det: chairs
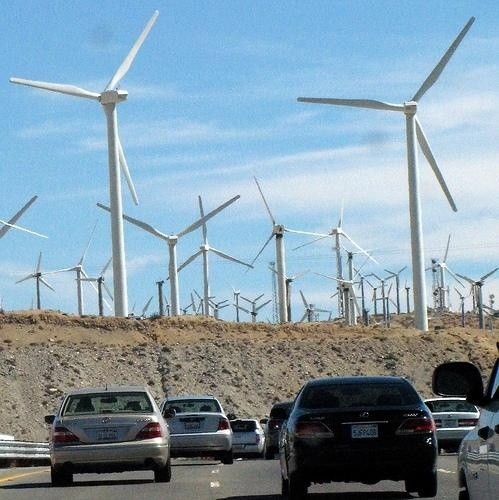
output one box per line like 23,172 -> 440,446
170,406 -> 181,412
74,403 -> 94,412
124,400 -> 142,410
200,405 -> 211,411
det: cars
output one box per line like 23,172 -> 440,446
260,357 -> 498,499
44,390 -> 265,484
